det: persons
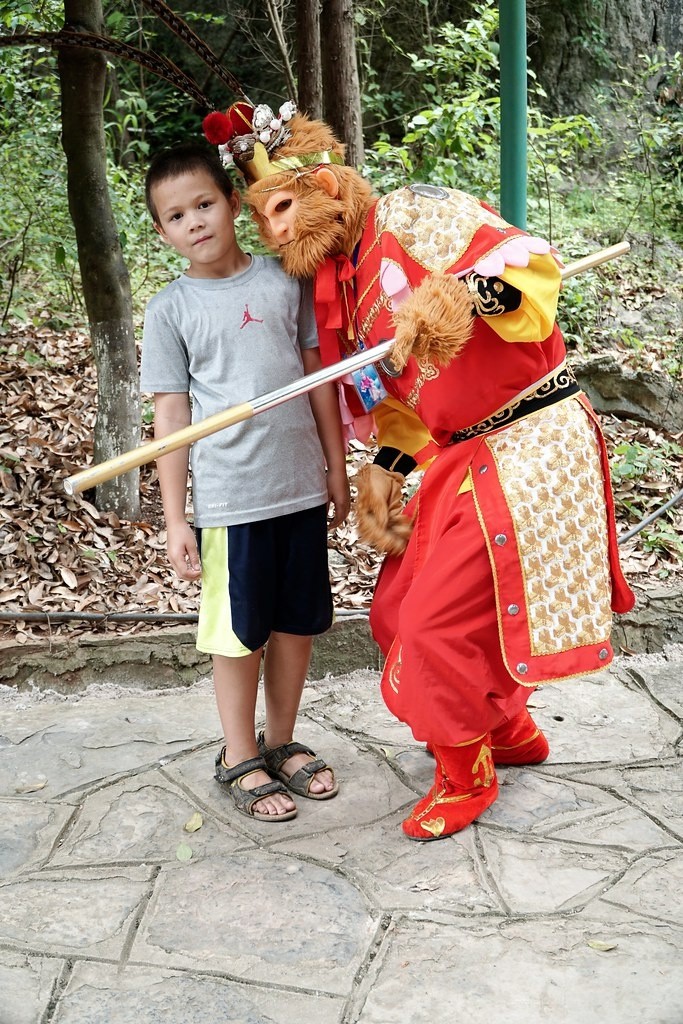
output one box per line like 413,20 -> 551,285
140,142 -> 351,822
203,104 -> 636,843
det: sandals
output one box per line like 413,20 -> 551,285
256,729 -> 339,801
214,745 -> 298,822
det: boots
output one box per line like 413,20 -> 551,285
403,733 -> 499,839
425,702 -> 550,768
